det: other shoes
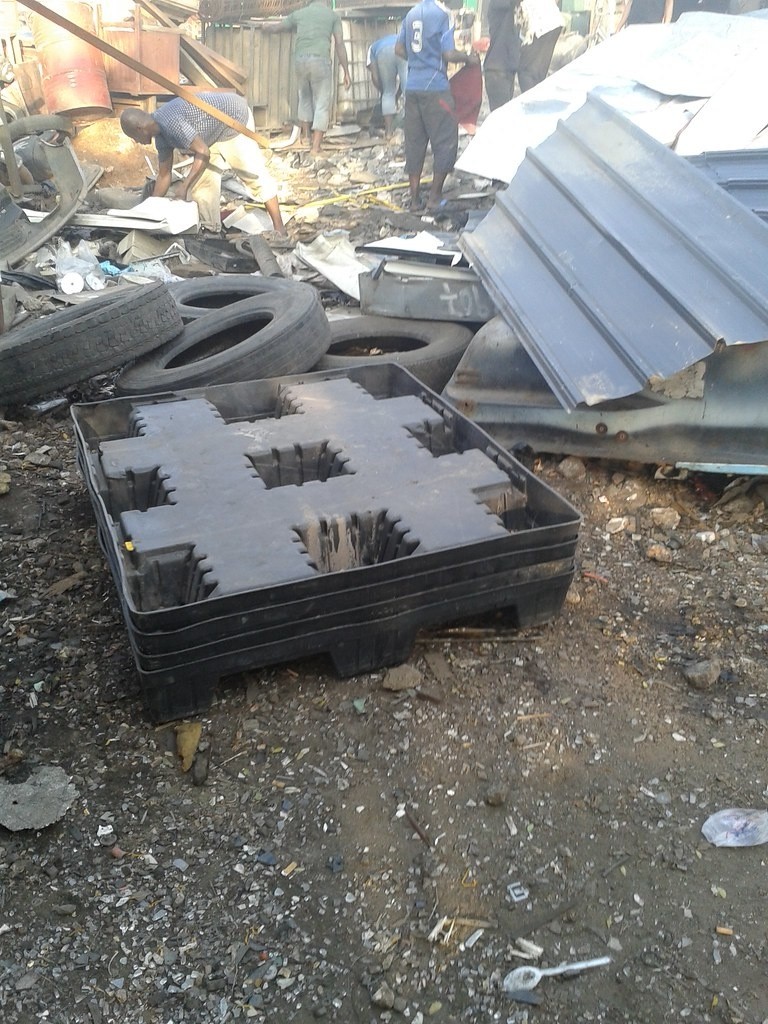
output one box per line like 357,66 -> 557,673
300,134 -> 313,146
309,150 -> 331,159
369,126 -> 391,140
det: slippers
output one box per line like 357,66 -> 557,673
426,200 -> 459,214
409,197 -> 428,211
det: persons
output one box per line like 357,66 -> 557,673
120,92 -> 283,230
365,36 -> 407,128
261,0 -> 352,150
615,0 -> 730,34
485,1 -> 562,111
395,0 -> 482,210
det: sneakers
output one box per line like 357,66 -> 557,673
267,230 -> 296,248
196,233 -> 223,241
